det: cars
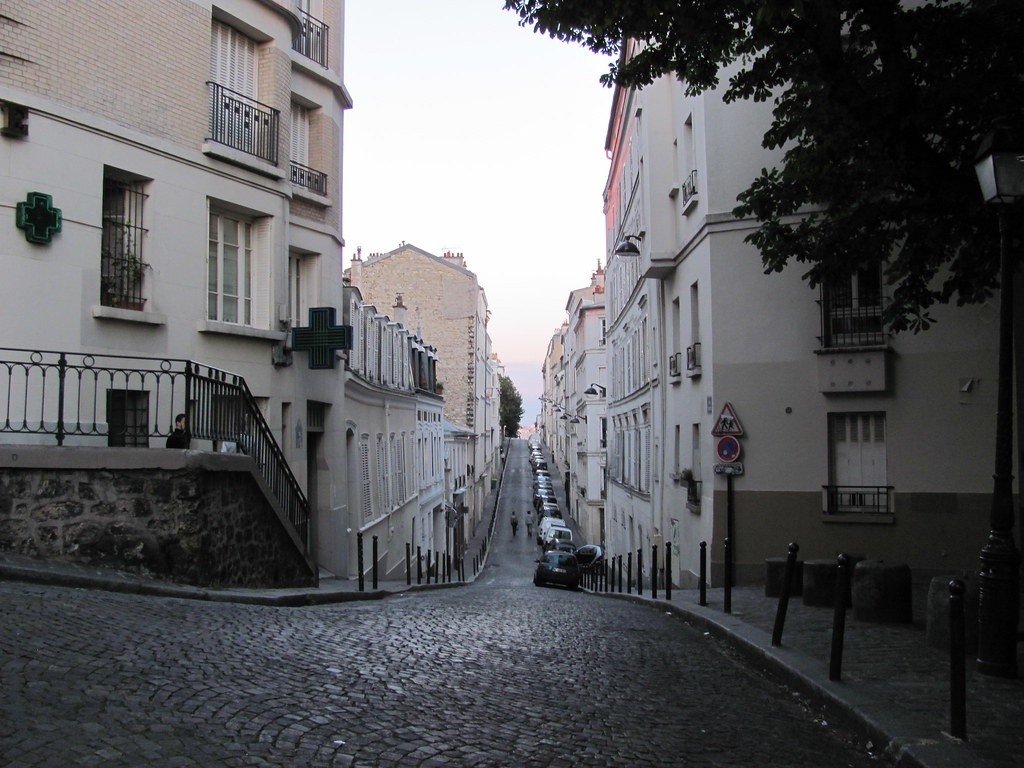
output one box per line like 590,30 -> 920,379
532,549 -> 584,592
573,544 -> 605,568
528,432 -> 577,554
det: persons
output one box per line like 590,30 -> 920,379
510,511 -> 518,536
525,510 -> 535,537
166,414 -> 189,450
221,414 -> 254,455
